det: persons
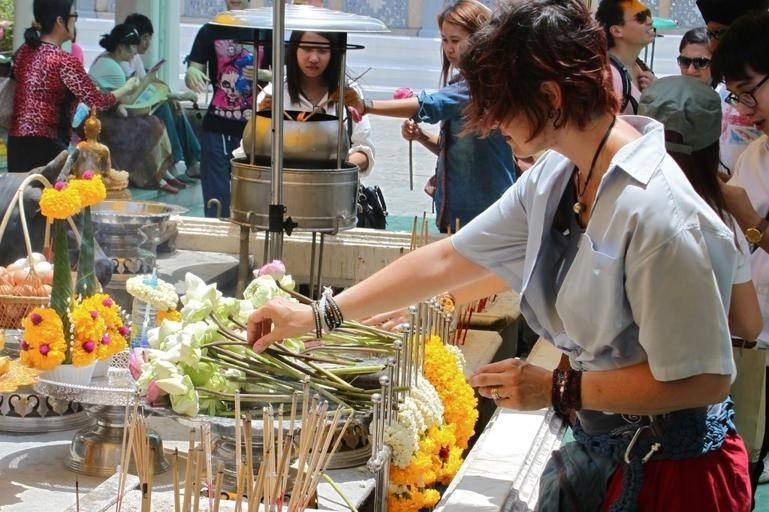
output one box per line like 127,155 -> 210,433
247,1 -> 752,511
5,0 -> 145,174
121,12 -> 205,186
83,23 -> 185,194
593,0 -> 658,117
258,30 -> 377,174
334,0 -> 517,238
638,0 -> 769,512
182,1 -> 281,221
73,104 -> 110,179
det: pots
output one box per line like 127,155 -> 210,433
242,110 -> 351,168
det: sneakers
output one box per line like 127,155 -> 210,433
169,178 -> 186,188
164,183 -> 176,192
186,171 -> 200,179
177,175 -> 196,183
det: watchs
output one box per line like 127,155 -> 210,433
744,219 -> 768,244
362,98 -> 373,114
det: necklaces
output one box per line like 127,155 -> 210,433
571,115 -> 618,215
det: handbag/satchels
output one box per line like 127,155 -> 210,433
357,182 -> 387,229
534,437 -> 623,512
424,176 -> 437,196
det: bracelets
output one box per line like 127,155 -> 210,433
553,352 -> 581,410
312,285 -> 343,338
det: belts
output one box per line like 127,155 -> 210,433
732,338 -> 760,348
580,414 -> 664,436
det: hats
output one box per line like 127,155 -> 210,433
639,76 -> 722,154
696,0 -> 768,27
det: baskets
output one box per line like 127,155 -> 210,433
1,174 -> 101,328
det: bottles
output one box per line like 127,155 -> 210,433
130,274 -> 164,353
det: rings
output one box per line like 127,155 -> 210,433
491,387 -> 502,402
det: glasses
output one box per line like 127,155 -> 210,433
705,28 -> 726,39
121,28 -> 140,43
633,9 -> 651,25
67,11 -> 78,21
725,76 -> 768,108
677,56 -> 710,70
